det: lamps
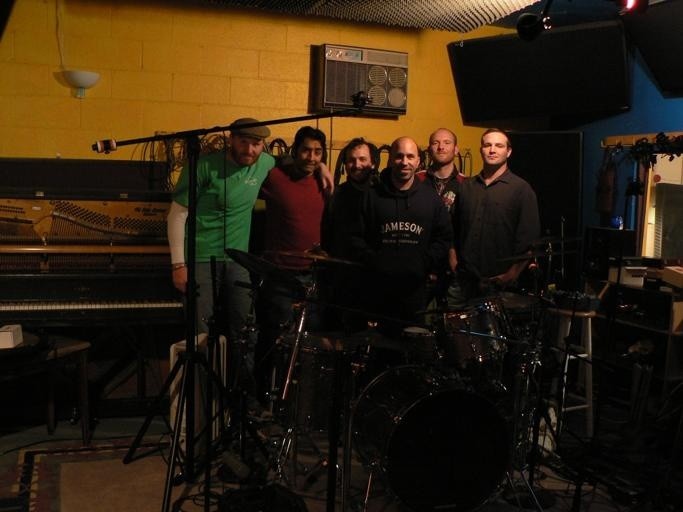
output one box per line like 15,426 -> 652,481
61,70 -> 100,99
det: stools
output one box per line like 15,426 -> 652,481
0,335 -> 92,448
533,308 -> 596,435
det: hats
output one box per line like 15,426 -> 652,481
229,118 -> 271,140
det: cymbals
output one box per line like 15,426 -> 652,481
225,249 -> 302,284
264,250 -> 353,264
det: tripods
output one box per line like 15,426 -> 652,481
250,249 -> 599,512
92,110 -> 357,512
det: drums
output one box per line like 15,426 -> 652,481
400,326 -> 436,364
350,365 -> 510,503
443,298 -> 501,363
295,349 -> 350,435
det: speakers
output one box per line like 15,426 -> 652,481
446,20 -> 631,127
621,0 -> 683,98
506,131 -> 585,240
321,43 -> 409,115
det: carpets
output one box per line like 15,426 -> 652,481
6,431 -> 630,511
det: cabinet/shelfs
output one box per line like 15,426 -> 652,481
583,267 -> 683,415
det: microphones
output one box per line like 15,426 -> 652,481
350,91 -> 373,109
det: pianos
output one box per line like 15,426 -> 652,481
0,158 -> 183,311
